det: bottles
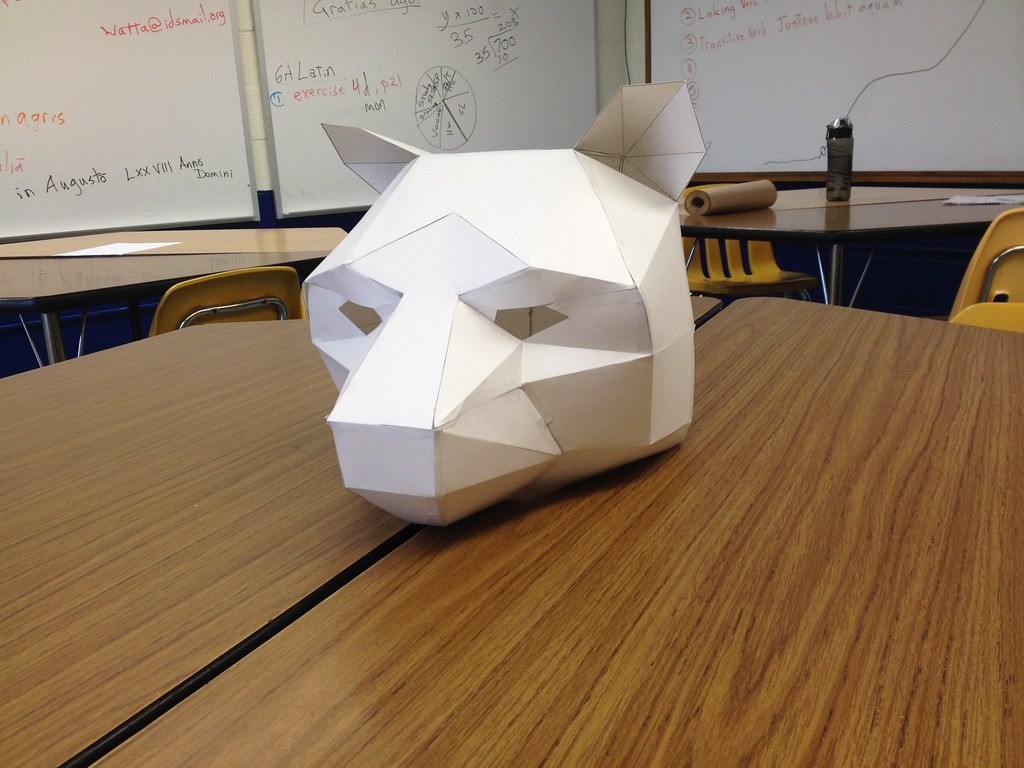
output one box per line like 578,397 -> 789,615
825,117 -> 854,201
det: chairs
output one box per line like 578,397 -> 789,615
673,176 -> 816,302
949,203 -> 1024,321
149,264 -> 299,340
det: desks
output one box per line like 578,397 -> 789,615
1,222 -> 352,374
2,289 -> 1024,767
676,180 -> 1024,321
